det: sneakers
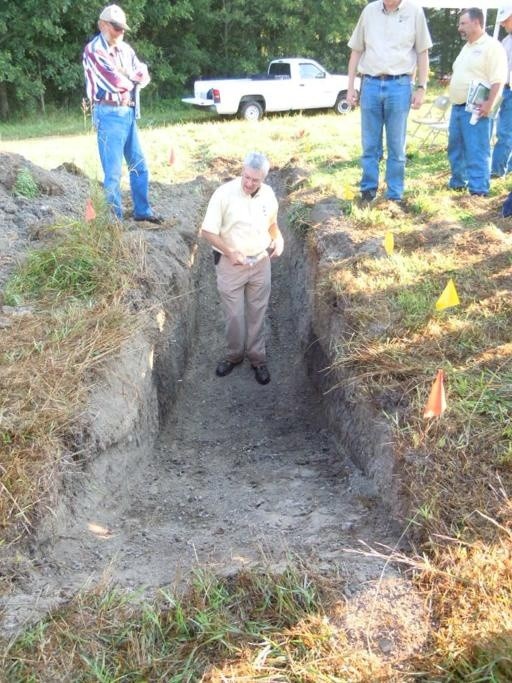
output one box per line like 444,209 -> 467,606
359,191 -> 375,210
134,211 -> 165,224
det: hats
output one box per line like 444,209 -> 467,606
496,0 -> 512,22
99,4 -> 131,30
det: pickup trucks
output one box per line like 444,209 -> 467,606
180,55 -> 362,120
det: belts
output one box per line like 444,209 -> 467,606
363,74 -> 407,80
100,99 -> 136,106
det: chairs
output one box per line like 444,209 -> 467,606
411,95 -> 451,147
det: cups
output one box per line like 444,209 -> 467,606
468,110 -> 481,126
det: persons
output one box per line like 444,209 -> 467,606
344,0 -> 434,202
199,151 -> 285,385
83,3 -> 166,224
441,7 -> 507,194
487,7 -> 512,178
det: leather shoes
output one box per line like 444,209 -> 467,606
216,359 -> 243,376
252,365 -> 269,385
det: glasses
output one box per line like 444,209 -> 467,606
110,24 -> 122,31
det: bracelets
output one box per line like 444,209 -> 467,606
415,84 -> 424,88
